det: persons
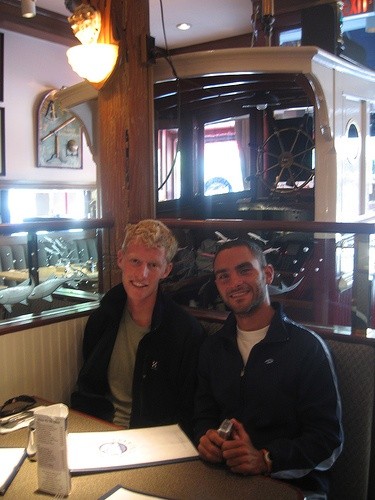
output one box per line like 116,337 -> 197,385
193,239 -> 344,476
71,219 -> 207,438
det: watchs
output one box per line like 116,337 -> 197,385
262,450 -> 272,474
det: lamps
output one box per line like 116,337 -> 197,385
21,0 -> 36,19
66,0 -> 119,83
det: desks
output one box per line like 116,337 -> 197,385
0,264 -> 101,289
0,395 -> 306,500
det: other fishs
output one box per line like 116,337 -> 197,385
0,273 -> 76,313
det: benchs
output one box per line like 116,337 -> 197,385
181,306 -> 375,500
0,229 -> 100,291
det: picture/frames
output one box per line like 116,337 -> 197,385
36,88 -> 84,169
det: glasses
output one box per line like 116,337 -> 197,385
0,395 -> 37,417
